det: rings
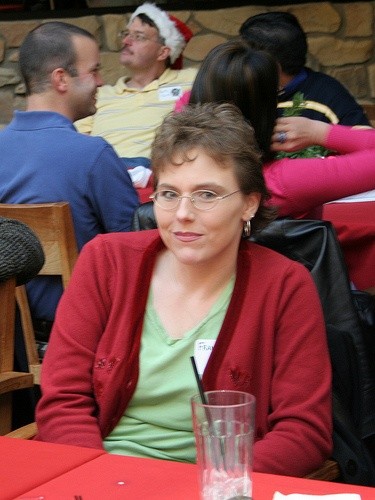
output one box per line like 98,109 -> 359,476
278,131 -> 286,144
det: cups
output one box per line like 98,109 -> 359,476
191,390 -> 256,500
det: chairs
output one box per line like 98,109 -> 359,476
0,200 -> 375,486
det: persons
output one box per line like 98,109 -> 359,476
0,3 -> 375,287
35,101 -> 332,479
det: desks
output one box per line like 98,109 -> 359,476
0,434 -> 375,500
128,166 -> 375,295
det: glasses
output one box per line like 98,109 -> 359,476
148,189 -> 250,210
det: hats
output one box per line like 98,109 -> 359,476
129,2 -> 193,69
